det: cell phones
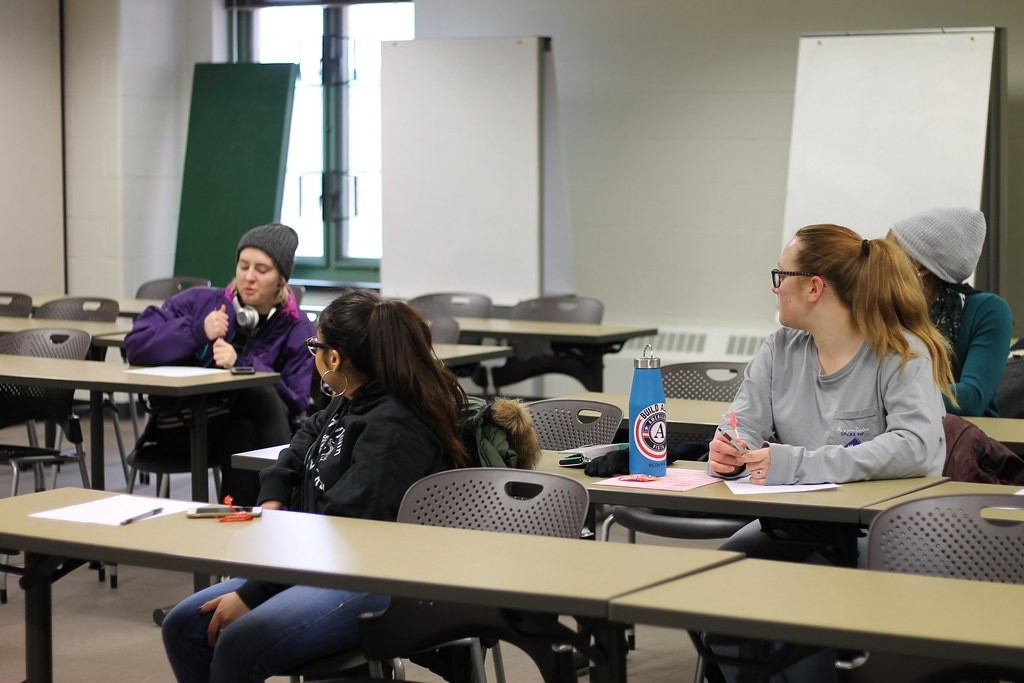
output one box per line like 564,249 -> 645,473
559,453 -> 583,465
187,506 -> 263,519
231,366 -> 254,375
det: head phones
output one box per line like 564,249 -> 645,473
232,290 -> 278,329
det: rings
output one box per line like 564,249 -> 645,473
757,470 -> 762,478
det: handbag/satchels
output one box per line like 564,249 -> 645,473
132,374 -> 258,472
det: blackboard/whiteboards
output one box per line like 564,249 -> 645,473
778,26 -> 996,302
380,35 -> 542,307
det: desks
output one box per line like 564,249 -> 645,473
860,481 -> 1024,528
231,439 -> 951,676
0,315 -> 134,470
0,291 -> 657,394
0,354 -> 281,626
0,298 -> 1024,565
1,484 -> 745,683
517,391 -> 1024,485
91,331 -> 514,397
608,558 -> 1024,683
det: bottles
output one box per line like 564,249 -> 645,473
628,344 -> 668,477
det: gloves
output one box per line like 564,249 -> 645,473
583,442 -> 681,477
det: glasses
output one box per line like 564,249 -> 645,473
306,335 -> 334,358
771,268 -> 827,291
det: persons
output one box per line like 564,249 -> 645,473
163,288 -> 468,683
708,224 -> 960,683
886,205 -> 1014,418
123,223 -> 316,505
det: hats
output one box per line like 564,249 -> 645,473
236,223 -> 299,280
890,206 -> 988,283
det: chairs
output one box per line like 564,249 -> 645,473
0,274 -> 1024,650
291,466 -> 589,683
836,493 -> 1023,683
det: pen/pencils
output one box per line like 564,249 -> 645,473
718,428 -> 746,453
730,410 -> 739,438
215,307 -> 221,339
119,508 -> 164,526
